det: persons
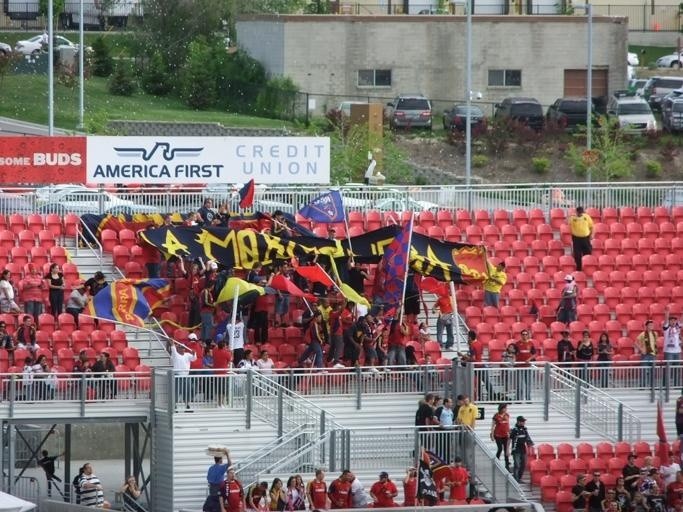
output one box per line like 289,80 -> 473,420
0,196 -> 682,511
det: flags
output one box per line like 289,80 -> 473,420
238,177 -> 254,208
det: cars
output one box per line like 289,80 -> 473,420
14,32 -> 94,63
0,41 -> 13,57
626,46 -> 683,136
336,99 -> 363,117
439,103 -> 487,133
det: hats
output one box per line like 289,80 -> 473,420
516,416 -> 525,421
563,275 -> 571,280
210,263 -> 218,269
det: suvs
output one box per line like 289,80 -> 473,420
488,94 -> 543,132
543,94 -> 599,130
383,92 -> 434,133
605,89 -> 659,138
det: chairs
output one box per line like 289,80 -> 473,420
365,496 -> 487,511
0,205 -> 682,387
525,440 -> 682,512
